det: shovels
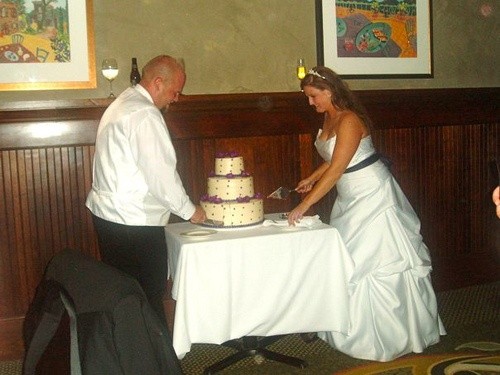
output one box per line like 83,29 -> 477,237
267,187 -> 295,200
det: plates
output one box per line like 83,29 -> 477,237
179,229 -> 216,241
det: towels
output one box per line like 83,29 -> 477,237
262,215 -> 322,229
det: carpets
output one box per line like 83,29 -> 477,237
330,353 -> 500,374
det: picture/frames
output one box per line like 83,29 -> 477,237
0,0 -> 98,92
315,0 -> 435,80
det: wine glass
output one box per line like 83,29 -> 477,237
102,59 -> 118,97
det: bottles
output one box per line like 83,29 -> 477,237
130,57 -> 140,86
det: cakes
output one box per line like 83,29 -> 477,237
199,151 -> 265,227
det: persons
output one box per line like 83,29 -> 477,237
288,64 -> 446,363
86,55 -> 207,343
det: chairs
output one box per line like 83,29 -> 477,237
37,250 -> 163,374
